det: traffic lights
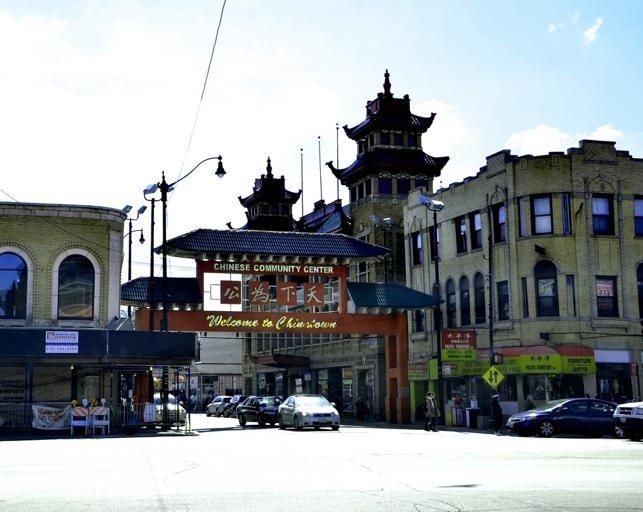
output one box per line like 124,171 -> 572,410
493,352 -> 503,365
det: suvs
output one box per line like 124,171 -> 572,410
612,402 -> 643,440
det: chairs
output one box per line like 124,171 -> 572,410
68,401 -> 112,438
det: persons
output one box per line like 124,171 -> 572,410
524,394 -> 535,411
356,396 -> 365,421
429,392 -> 438,432
424,392 -> 438,432
491,395 -> 504,435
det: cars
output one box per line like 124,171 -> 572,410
504,398 -> 620,438
141,391 -> 187,429
205,388 -> 340,431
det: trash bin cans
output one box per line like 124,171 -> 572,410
466,407 -> 480,428
477,413 -> 489,429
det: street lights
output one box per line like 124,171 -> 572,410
369,212 -> 393,284
413,194 -> 446,425
120,153 -> 226,427
